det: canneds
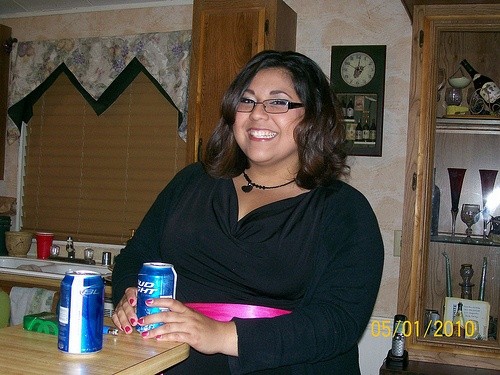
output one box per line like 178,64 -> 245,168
58,270 -> 105,354
136,262 -> 177,334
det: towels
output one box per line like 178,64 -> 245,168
9,286 -> 56,326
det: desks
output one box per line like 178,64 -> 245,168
0,323 -> 189,375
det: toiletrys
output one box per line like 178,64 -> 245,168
129,228 -> 135,240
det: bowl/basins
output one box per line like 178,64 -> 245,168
5,231 -> 34,256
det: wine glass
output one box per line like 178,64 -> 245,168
447,167 -> 467,237
478,168 -> 498,239
460,204 -> 480,239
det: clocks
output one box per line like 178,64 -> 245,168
329,45 -> 387,157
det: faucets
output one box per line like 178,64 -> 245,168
66,236 -> 76,259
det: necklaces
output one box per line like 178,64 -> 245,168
242,171 -> 296,193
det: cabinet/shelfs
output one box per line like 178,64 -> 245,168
398,0 -> 500,371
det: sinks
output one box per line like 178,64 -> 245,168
0,256 -> 109,280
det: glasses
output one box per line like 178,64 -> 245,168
236,99 -> 303,113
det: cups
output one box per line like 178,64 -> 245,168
36,232 -> 55,260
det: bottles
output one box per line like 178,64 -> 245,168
430,310 -> 441,334
460,58 -> 500,114
355,118 -> 363,141
346,99 -> 355,119
450,302 -> 465,339
369,118 -> 376,142
427,168 -> 441,237
340,99 -> 347,119
362,118 -> 370,142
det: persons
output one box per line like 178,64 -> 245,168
110,50 -> 385,375
